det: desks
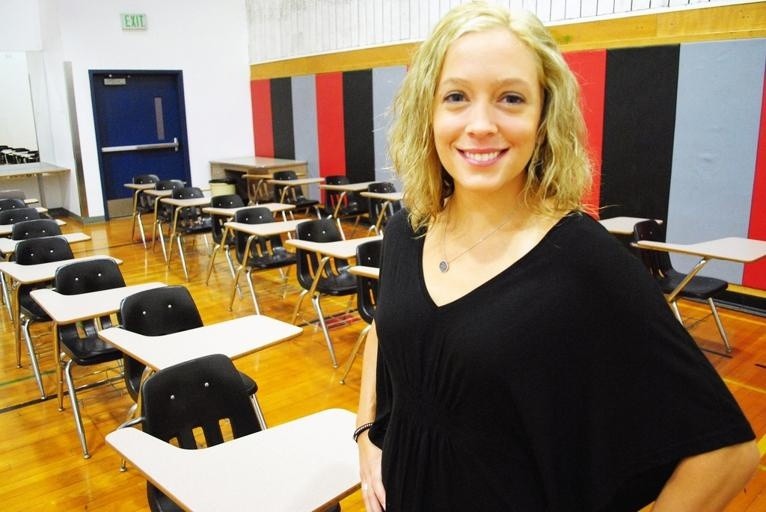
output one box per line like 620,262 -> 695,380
0,161 -> 70,207
208,157 -> 309,200
628,236 -> 765,304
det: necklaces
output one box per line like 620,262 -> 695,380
436,192 -> 529,275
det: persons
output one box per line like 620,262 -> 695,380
349,0 -> 761,512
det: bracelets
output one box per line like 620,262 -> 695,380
351,423 -> 377,441
